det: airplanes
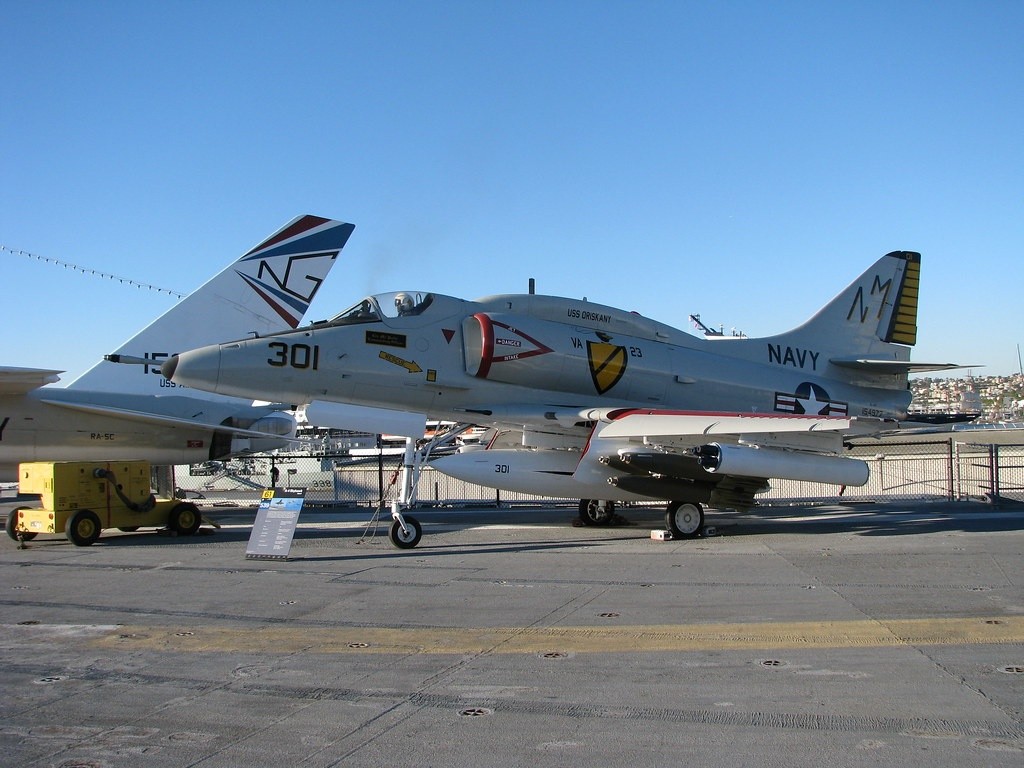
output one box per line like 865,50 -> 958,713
0,212 -> 1023,550
